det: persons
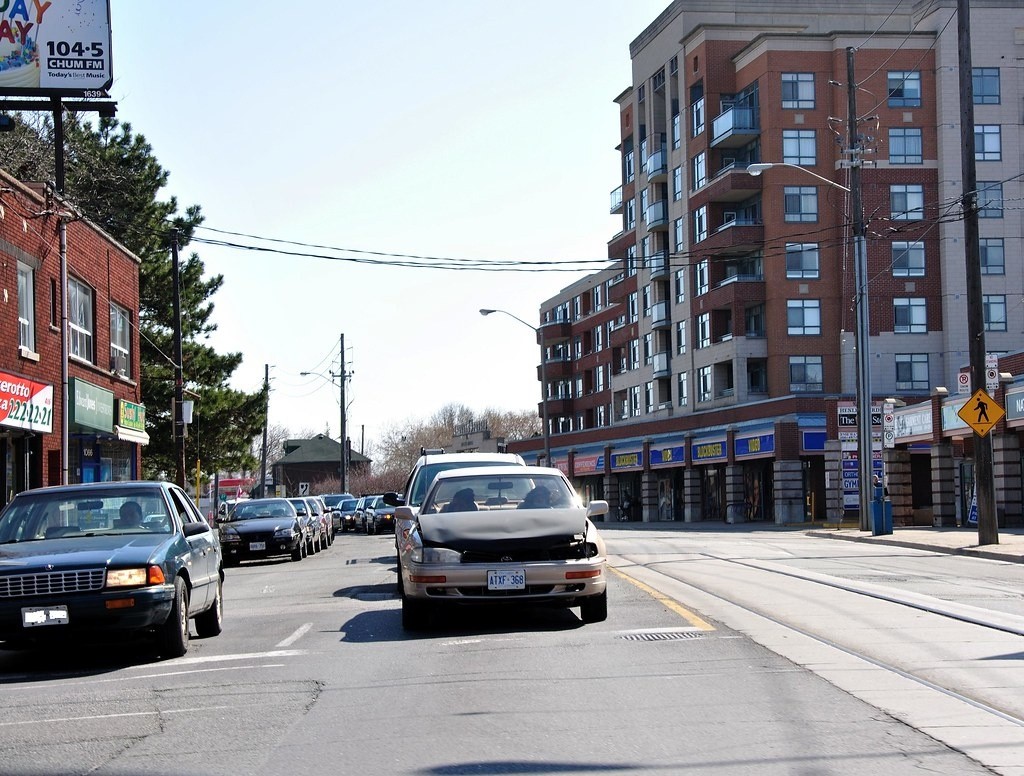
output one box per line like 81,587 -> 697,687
621,489 -> 631,521
119,501 -> 143,528
440,487 -> 552,513
873,474 -> 888,496
748,473 -> 764,518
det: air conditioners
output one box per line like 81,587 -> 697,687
110,357 -> 126,370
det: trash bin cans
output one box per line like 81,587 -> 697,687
869,487 -> 893,536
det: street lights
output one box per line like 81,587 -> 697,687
478,309 -> 551,469
300,371 -> 347,495
747,161 -> 873,529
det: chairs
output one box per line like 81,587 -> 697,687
440,502 -> 478,512
141,521 -> 166,532
241,513 -> 256,519
368,502 -> 372,507
45,527 -> 81,538
378,503 -> 386,509
272,510 -> 284,516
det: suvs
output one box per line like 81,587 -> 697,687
382,452 -> 561,548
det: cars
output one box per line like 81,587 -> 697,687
0,478 -> 226,664
216,491 -> 408,567
395,465 -> 610,629
